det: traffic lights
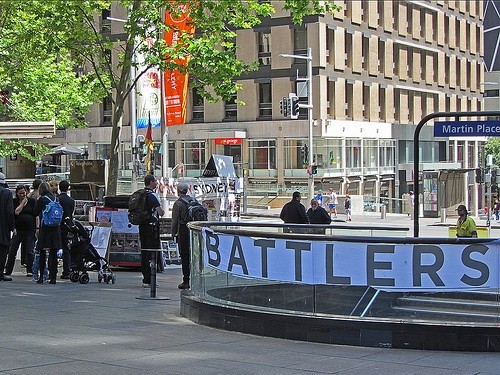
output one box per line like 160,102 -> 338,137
291,96 -> 300,119
280,96 -> 287,117
312,165 -> 318,174
475,168 -> 481,183
139,152 -> 147,159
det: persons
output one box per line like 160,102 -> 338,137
280,191 -> 351,234
0,172 -> 76,284
455,205 -> 478,238
408,192 -> 415,221
485,199 -> 500,225
138,175 -> 165,288
172,182 -> 198,289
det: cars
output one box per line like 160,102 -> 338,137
164,164 -> 203,178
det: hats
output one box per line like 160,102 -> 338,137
454,204 -> 466,211
0,172 -> 6,184
176,183 -> 189,192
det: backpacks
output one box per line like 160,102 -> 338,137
180,196 -> 208,221
128,189 -> 156,225
41,195 -> 64,227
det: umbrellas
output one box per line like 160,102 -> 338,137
46,141 -> 84,172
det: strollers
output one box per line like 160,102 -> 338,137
61,217 -> 116,285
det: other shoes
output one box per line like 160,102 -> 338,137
36,279 -> 43,284
0,276 -> 13,281
60,273 -> 70,279
3,271 -> 10,275
142,282 -> 159,288
178,281 -> 190,289
49,280 -> 56,284
